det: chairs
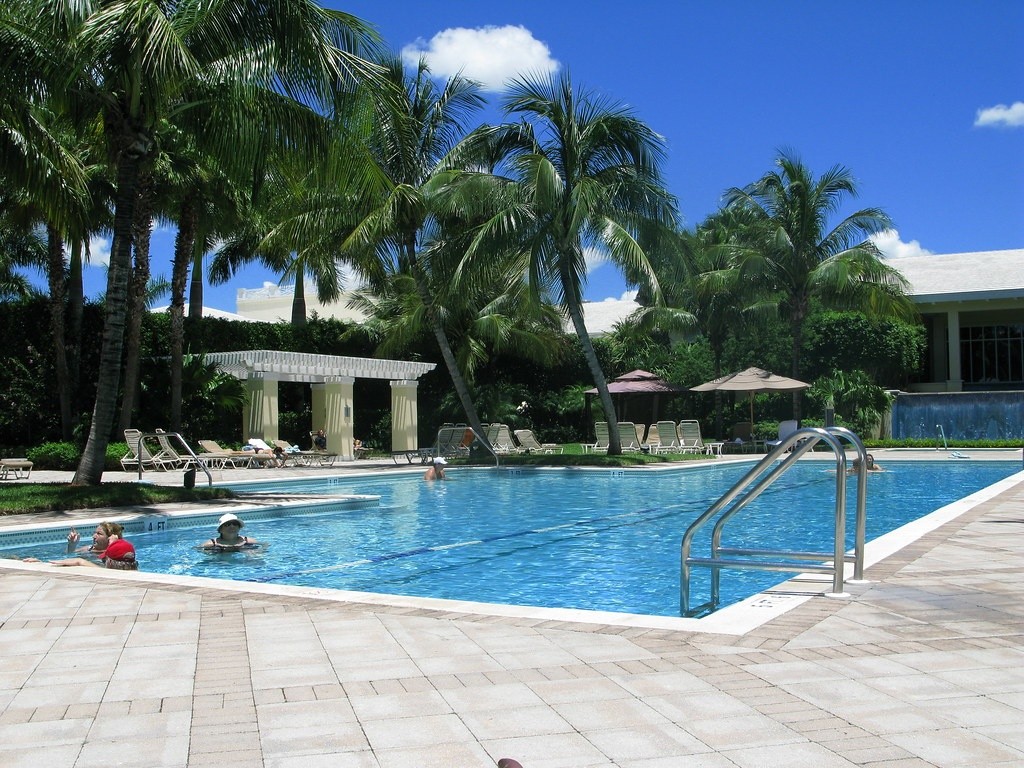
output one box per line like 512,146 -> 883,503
635,424 -> 645,446
249,438 -> 321,467
418,423 -> 518,465
593,421 -> 609,454
655,421 -> 679,455
271,440 -> 337,467
679,420 -> 706,455
121,429 -> 170,473
309,431 -> 320,452
514,430 -> 564,455
725,423 -> 751,455
156,429 -> 229,471
354,448 -> 374,460
646,423 -> 660,454
198,440 -> 270,471
766,421 -> 798,452
617,422 -> 641,453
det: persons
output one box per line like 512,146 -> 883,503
424,457 -> 448,480
315,428 -> 362,451
67,522 -> 124,552
202,513 -> 254,545
252,446 -> 288,468
23,539 -> 138,570
852,454 -> 879,471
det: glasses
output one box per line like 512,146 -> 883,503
222,521 -> 241,527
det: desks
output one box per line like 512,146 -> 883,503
580,444 -> 596,454
391,450 -> 419,464
752,439 -> 768,453
705,443 -> 724,455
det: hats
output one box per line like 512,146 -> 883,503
434,457 -> 448,464
216,513 -> 244,531
98,539 -> 135,561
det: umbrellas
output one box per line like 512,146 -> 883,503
582,369 -> 688,422
688,367 -> 812,439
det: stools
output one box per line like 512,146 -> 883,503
0,459 -> 33,480
